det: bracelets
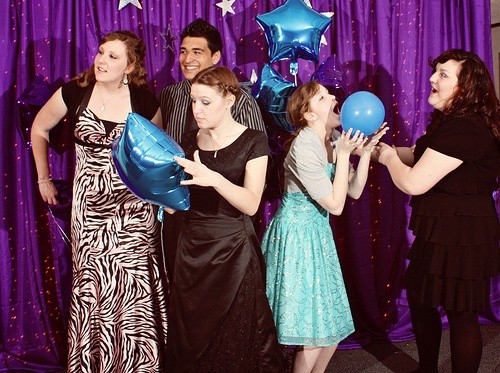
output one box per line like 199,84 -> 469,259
37,178 -> 52,183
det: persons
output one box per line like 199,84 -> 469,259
31,30 -> 170,373
165,67 -> 283,373
161,17 -> 267,142
261,82 -> 389,373
350,49 -> 500,373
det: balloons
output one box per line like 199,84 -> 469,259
255,0 -> 333,64
340,92 -> 385,136
255,64 -> 297,133
113,113 -> 190,210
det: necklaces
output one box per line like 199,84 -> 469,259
208,120 -> 236,158
96,83 -> 124,110
